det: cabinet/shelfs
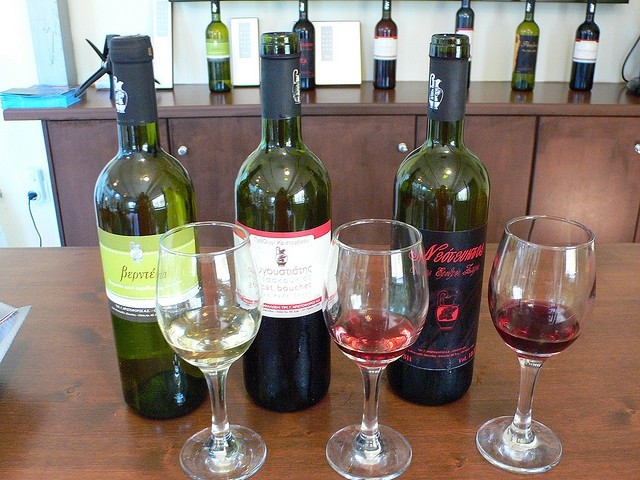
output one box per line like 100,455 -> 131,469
0,84 -> 640,246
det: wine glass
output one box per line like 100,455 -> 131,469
157,222 -> 267,476
324,218 -> 430,480
477,213 -> 598,473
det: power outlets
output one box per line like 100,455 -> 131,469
26,169 -> 46,205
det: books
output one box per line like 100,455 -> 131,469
0,84 -> 81,108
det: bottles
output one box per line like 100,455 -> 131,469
234,31 -> 334,412
455,0 -> 474,88
569,0 -> 600,92
390,33 -> 489,403
205,1 -> 233,93
511,1 -> 540,92
293,0 -> 316,91
372,1 -> 397,87
95,34 -> 207,416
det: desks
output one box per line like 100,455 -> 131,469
0,241 -> 640,480
